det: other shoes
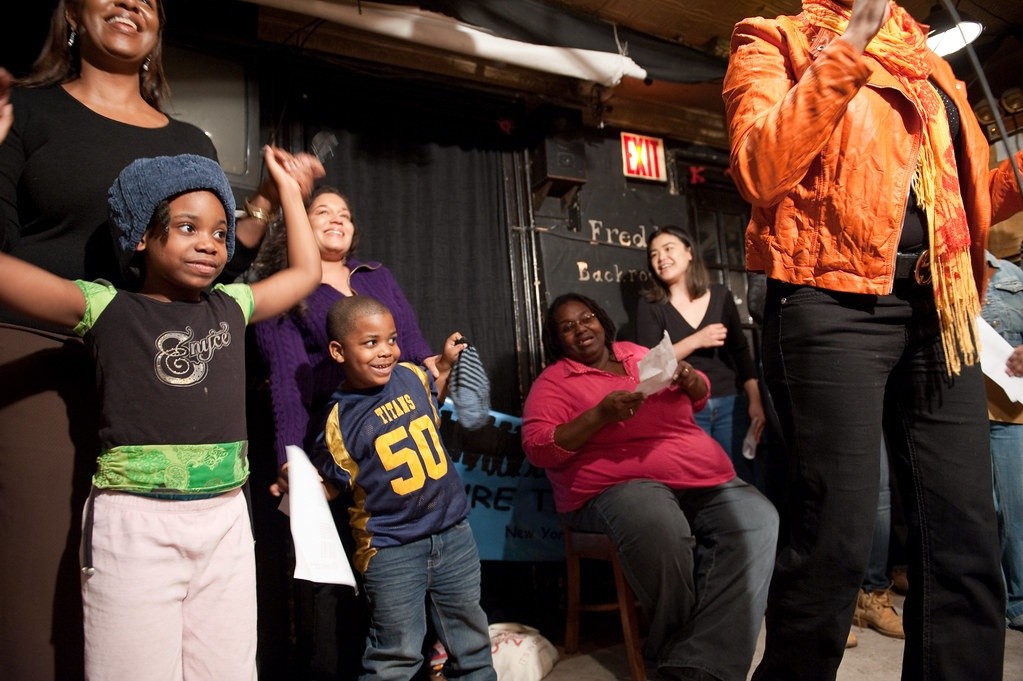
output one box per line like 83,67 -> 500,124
1008,623 -> 1022,629
890,570 -> 908,592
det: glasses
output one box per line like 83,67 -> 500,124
559,313 -> 598,336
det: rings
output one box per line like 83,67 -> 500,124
685,369 -> 689,373
629,408 -> 634,416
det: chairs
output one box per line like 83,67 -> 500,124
559,513 -> 697,680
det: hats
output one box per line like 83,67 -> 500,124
448,345 -> 490,430
106,152 -> 236,277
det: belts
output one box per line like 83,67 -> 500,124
895,250 -> 933,286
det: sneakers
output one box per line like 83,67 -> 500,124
845,633 -> 856,645
853,590 -> 905,639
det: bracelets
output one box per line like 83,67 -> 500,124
243,196 -> 270,223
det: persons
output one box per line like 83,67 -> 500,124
723,0 -> 1023,681
520,293 -> 780,681
637,225 -> 766,463
0,0 -> 328,681
251,186 -> 499,681
